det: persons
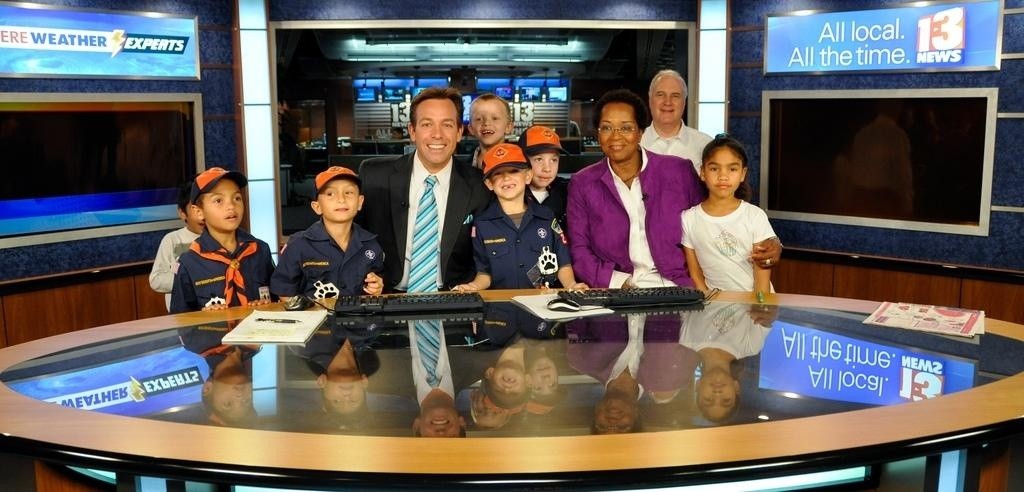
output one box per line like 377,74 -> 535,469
287,304 -> 780,440
169,167 -> 280,313
449,145 -> 590,295
831,101 -> 915,218
640,68 -> 715,177
565,89 -> 784,290
679,133 -> 783,294
354,87 -> 494,293
519,125 -> 571,288
149,181 -> 206,315
177,321 -> 264,429
465,94 -> 515,170
268,166 -> 384,311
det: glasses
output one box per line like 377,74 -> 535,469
598,126 -> 637,135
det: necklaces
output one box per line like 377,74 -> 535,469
620,151 -> 643,183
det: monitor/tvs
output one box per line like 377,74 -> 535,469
377,142 -> 404,154
354,85 -> 569,128
759,86 -> 999,239
558,139 -> 580,155
457,140 -> 478,154
0,91 -> 208,252
349,141 -> 376,154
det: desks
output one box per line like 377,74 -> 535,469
0,287 -> 1024,491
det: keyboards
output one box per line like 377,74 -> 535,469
333,291 -> 487,313
558,285 -> 707,307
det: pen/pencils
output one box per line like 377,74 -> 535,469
254,317 -> 301,323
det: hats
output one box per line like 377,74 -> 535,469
484,391 -> 525,414
525,382 -> 568,414
520,125 -> 571,156
191,167 -> 248,205
314,166 -> 360,200
202,397 -> 257,426
482,142 -> 532,179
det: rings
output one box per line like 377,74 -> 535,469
766,259 -> 772,265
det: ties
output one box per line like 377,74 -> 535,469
408,176 -> 438,293
414,318 -> 441,390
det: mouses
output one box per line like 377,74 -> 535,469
548,298 -> 581,312
284,294 -> 315,312
